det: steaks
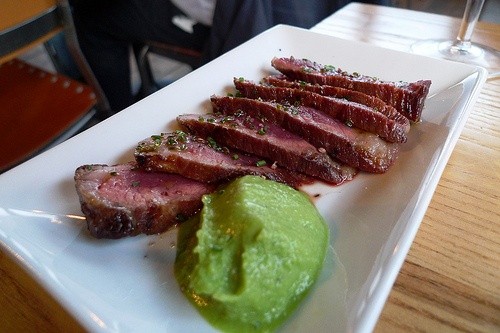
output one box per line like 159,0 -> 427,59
72,55 -> 431,238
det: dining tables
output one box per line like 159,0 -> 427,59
0,0 -> 500,332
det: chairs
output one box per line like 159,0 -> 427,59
1,1 -> 111,174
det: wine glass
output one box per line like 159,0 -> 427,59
407,0 -> 500,78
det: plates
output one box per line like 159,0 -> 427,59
1,23 -> 488,332
